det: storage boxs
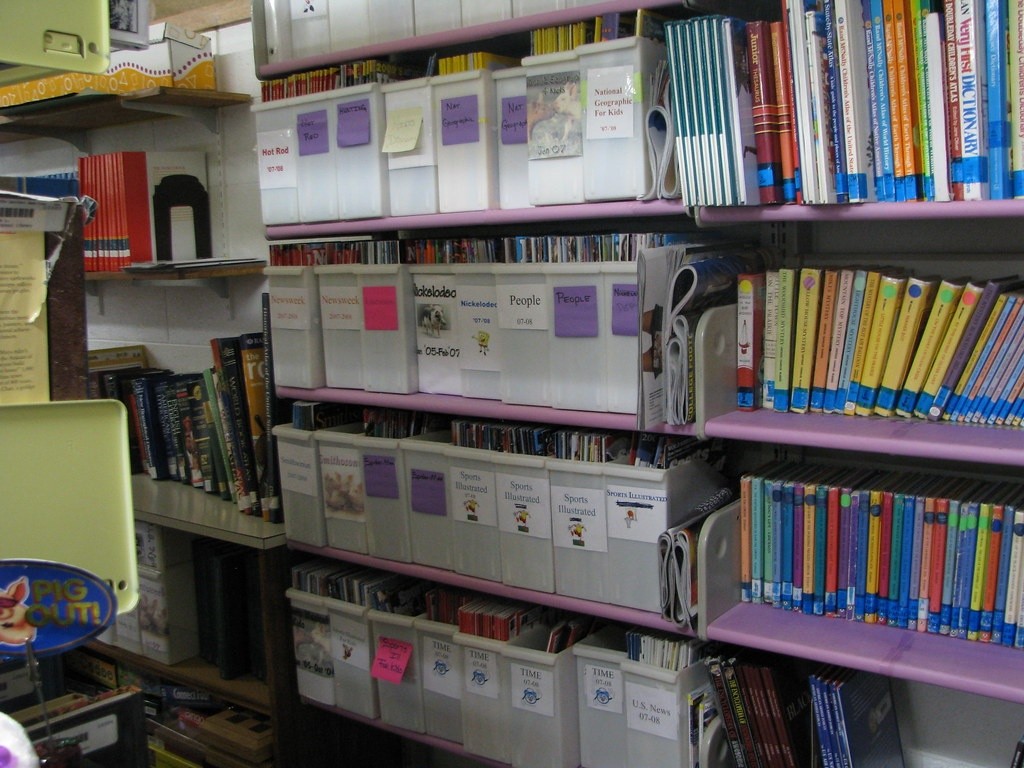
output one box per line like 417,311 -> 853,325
1,173 -> 95,405
1,23 -> 218,108
263,0 -> 615,65
267,261 -> 643,414
96,521 -> 280,768
250,38 -> 671,226
285,588 -> 706,768
274,425 -> 720,614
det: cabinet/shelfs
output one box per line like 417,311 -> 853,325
251,0 -> 1024,767
0,1 -> 148,768
0,86 -> 287,768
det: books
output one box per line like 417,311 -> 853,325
0,0 -> 1024,768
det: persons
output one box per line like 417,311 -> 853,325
643,304 -> 663,379
